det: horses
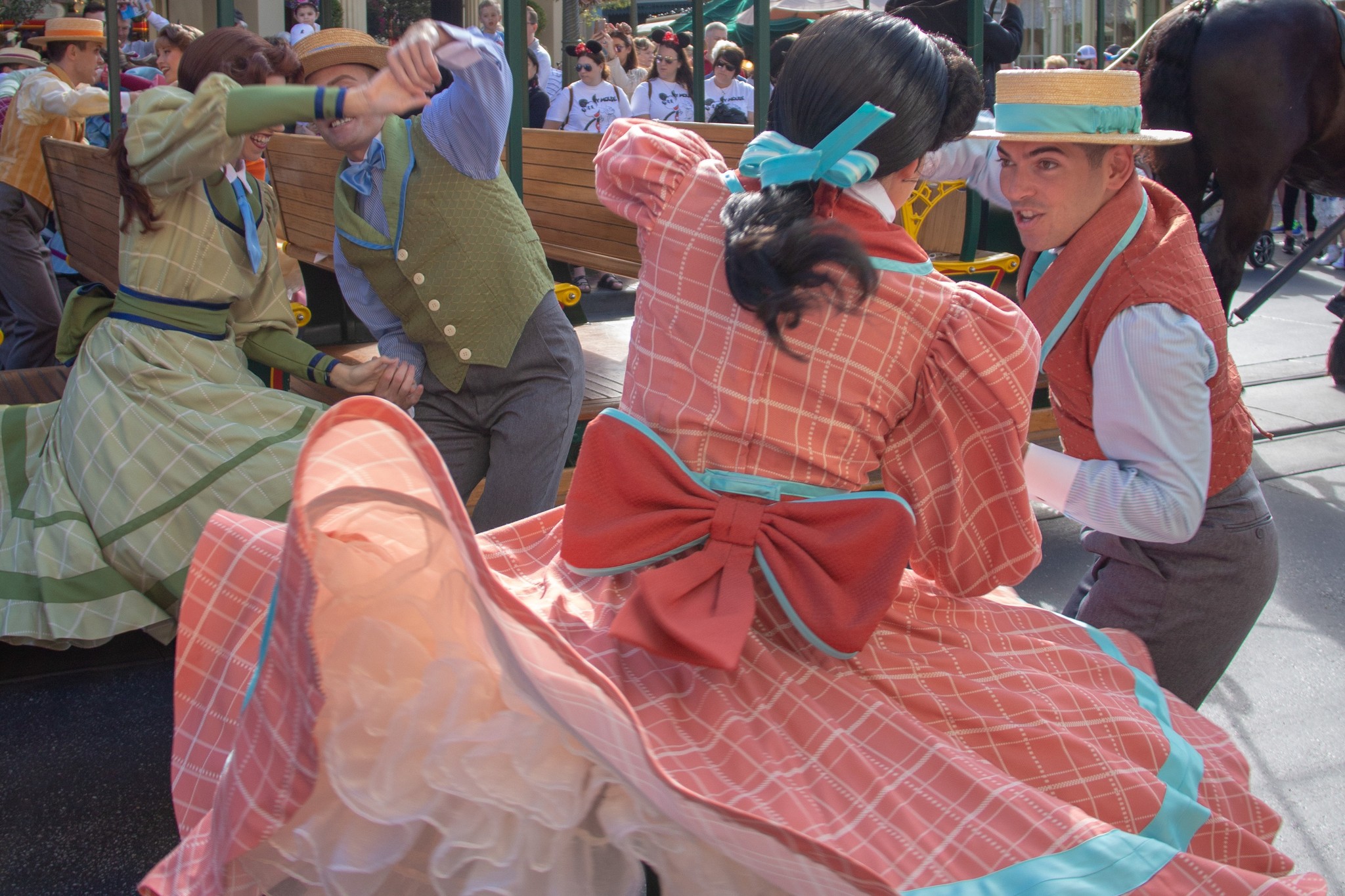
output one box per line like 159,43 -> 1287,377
1137,0 -> 1345,322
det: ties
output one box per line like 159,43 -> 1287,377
231,177 -> 262,274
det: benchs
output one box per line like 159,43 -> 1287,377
493,124 -> 1058,437
259,126 -> 632,420
36,131 -> 382,410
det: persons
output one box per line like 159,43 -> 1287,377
1263,174 -> 1345,271
132,9 -> 1323,895
884,0 -> 1139,116
915,69 -> 1279,711
463,0 -> 774,135
288,0 -> 322,49
0,18 -> 147,370
281,18 -> 586,536
93,23 -> 204,91
0,0 -> 173,149
0,27 -> 430,649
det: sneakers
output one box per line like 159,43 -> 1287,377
1283,236 -> 1295,254
1332,248 -> 1345,269
1316,244 -> 1341,265
1300,237 -> 1323,256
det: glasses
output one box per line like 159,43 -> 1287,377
715,60 -> 737,71
1122,58 -> 1137,65
655,54 -> 679,64
614,46 -> 629,53
574,64 -> 600,72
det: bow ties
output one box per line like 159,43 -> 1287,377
338,138 -> 386,196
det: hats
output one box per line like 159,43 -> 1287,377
960,68 -> 1193,145
1114,47 -> 1139,61
1075,45 -> 1097,58
290,23 -> 315,46
289,28 -> 393,79
27,17 -> 122,48
0,47 -> 48,68
